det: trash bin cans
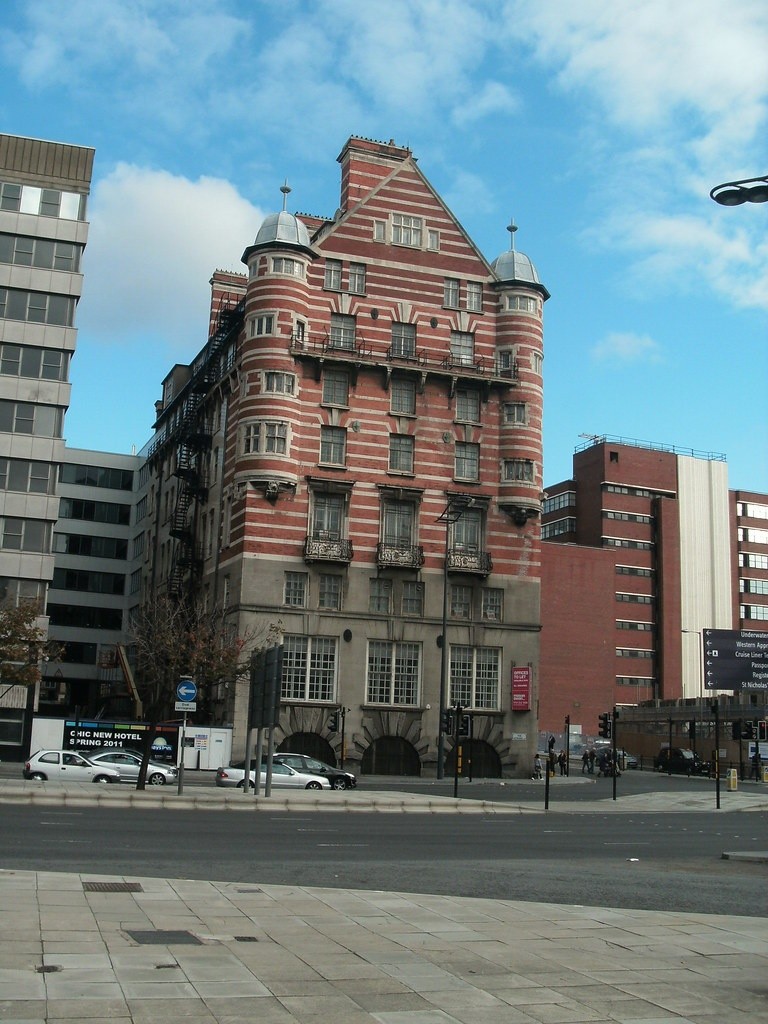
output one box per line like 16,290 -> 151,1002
617,751 -> 628,770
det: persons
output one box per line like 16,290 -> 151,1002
582,748 -> 622,777
749,754 -> 762,780
66,756 -> 77,765
549,749 -> 567,777
532,754 -> 544,780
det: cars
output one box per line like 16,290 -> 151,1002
654,746 -> 710,776
260,752 -> 357,791
216,757 -> 331,791
75,748 -> 179,786
594,745 -> 638,769
22,748 -> 121,784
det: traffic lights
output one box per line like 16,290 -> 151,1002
328,712 -> 337,732
598,712 -> 611,738
441,709 -> 453,737
459,715 -> 469,738
